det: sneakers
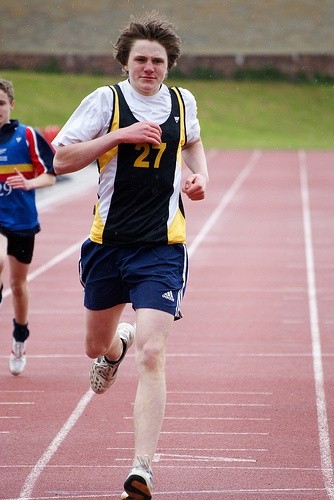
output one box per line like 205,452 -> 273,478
8,336 -> 26,378
88,321 -> 135,395
119,455 -> 154,500
